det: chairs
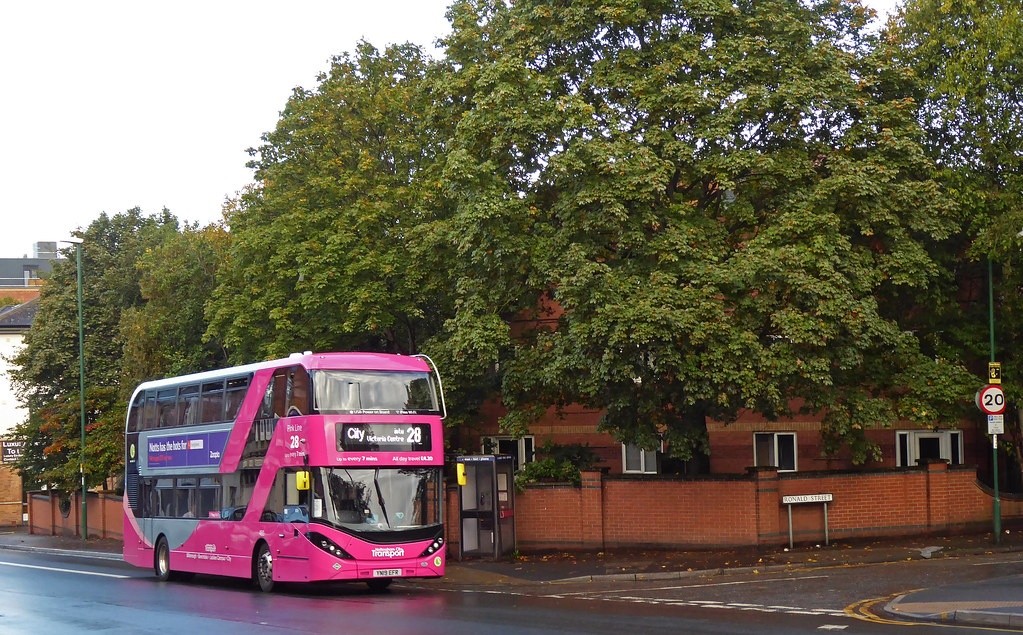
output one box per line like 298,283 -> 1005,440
159,403 -> 191,428
166,503 -> 173,516
228,506 -> 277,521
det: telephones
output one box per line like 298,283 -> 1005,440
480,491 -> 492,506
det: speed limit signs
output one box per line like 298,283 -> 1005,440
978,386 -> 1007,414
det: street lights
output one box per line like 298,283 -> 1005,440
59,236 -> 88,540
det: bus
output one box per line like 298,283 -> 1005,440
121,352 -> 470,593
